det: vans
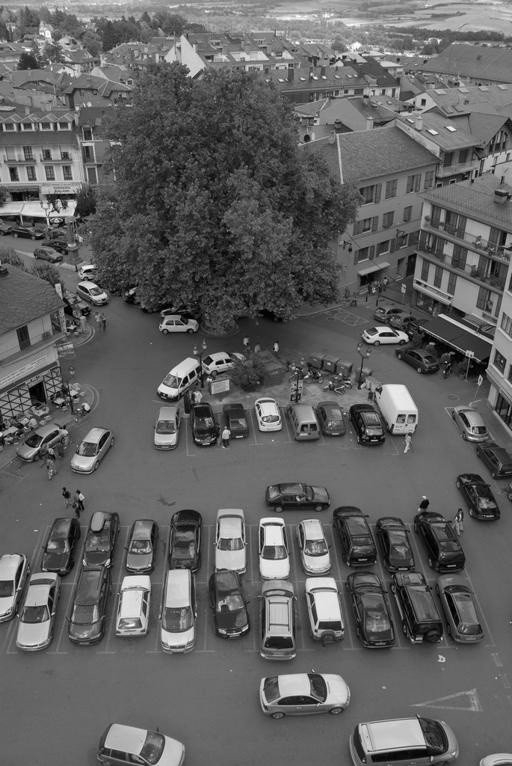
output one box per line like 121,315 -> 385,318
157,357 -> 204,402
374,381 -> 419,436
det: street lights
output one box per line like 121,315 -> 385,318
64,365 -> 77,415
355,342 -> 373,389
289,357 -> 306,402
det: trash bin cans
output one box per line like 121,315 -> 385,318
81,317 -> 88,334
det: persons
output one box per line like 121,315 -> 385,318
242,335 -> 280,354
402,432 -> 412,453
100,313 -> 106,329
0,416 -> 31,445
453,509 -> 464,536
61,486 -> 85,519
95,311 -> 101,329
367,276 -> 389,295
418,496 -> 429,512
222,426 -> 231,448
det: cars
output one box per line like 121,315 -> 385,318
258,669 -> 351,720
201,350 -> 246,377
16,423 -> 69,463
452,405 -> 512,521
266,482 -> 331,512
349,712 -> 459,766
318,400 -> 347,437
362,304 -> 440,375
478,752 -> 511,766
0,218 -> 202,335
69,427 -> 116,475
223,402 -> 249,440
255,396 -> 283,433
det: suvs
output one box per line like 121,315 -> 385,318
190,402 -> 218,446
286,403 -> 321,442
152,406 -> 182,451
96,722 -> 185,766
350,403 -> 385,447
